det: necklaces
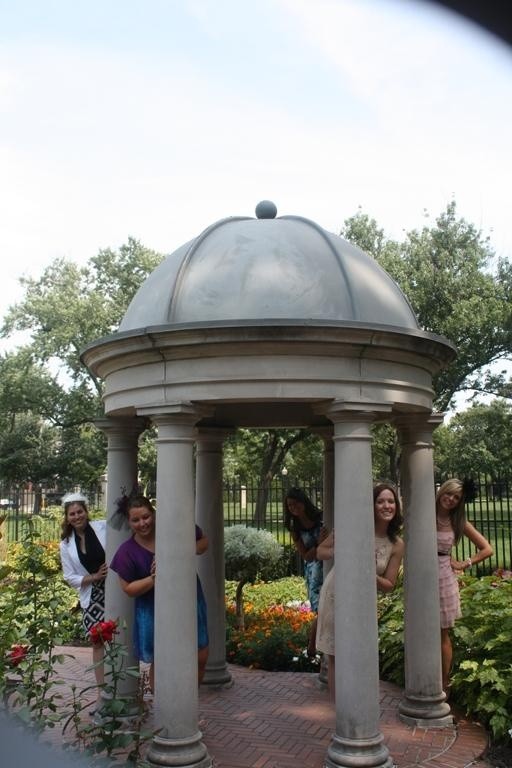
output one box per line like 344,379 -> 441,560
435,513 -> 451,528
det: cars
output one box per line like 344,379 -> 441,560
44,492 -> 64,507
0,497 -> 20,510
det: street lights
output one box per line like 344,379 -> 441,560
282,467 -> 288,496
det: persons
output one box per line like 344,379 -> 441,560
107,487 -> 211,742
283,487 -> 329,658
59,492 -> 108,715
315,484 -> 405,696
435,476 -> 496,704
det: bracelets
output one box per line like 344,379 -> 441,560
459,559 -> 463,569
312,541 -> 319,548
465,556 -> 473,568
90,572 -> 96,583
